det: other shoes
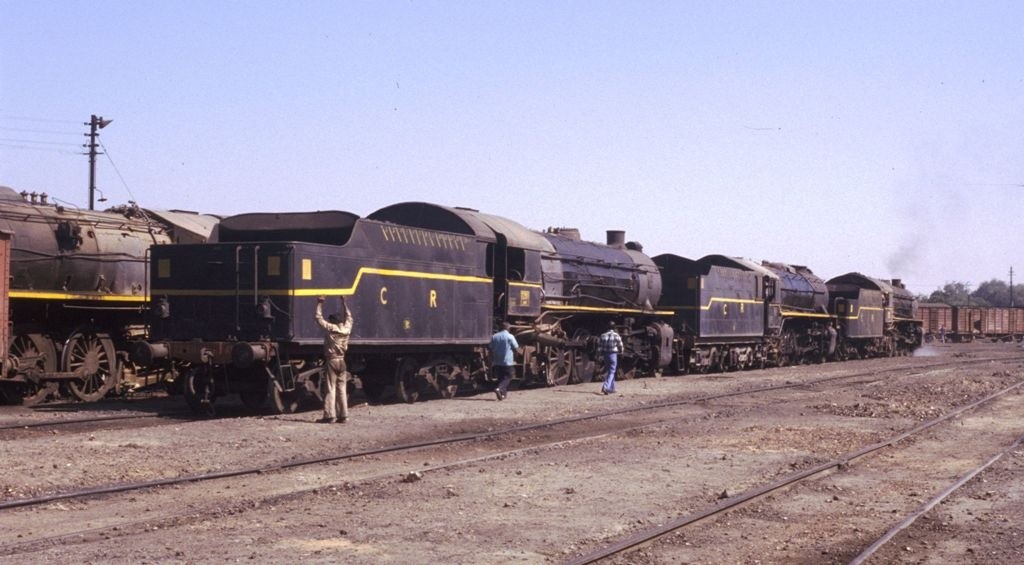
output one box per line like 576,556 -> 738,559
609,389 -> 617,393
337,416 -> 348,422
316,418 -> 337,423
494,387 -> 502,400
501,390 -> 506,399
602,387 -> 608,395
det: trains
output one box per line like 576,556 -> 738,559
0,186 -> 1024,415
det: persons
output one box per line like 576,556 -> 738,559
313,294 -> 353,423
598,321 -> 625,394
489,321 -> 525,401
